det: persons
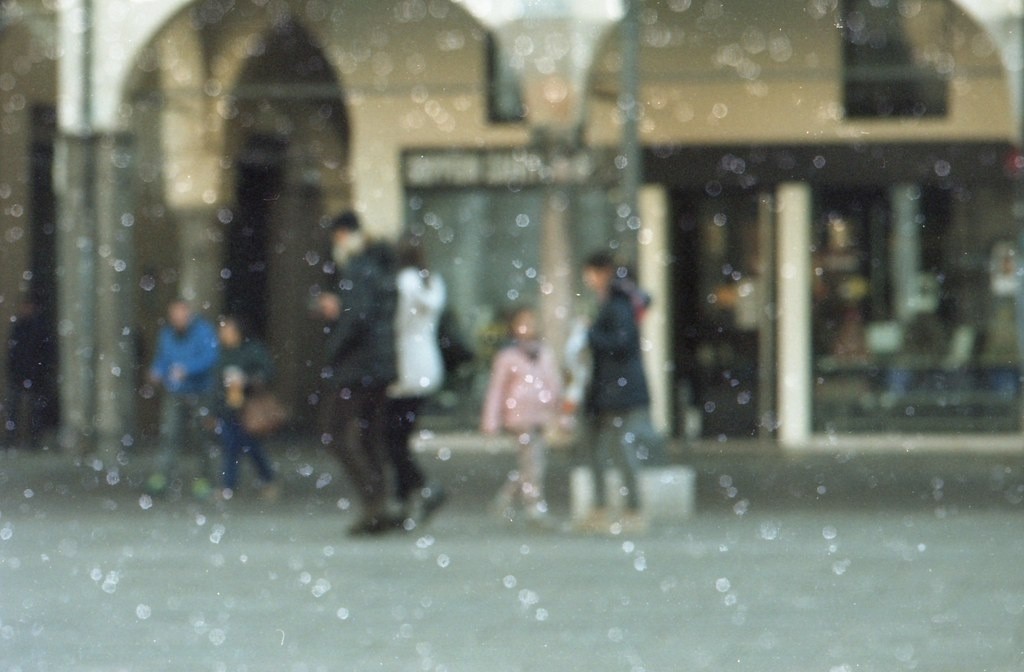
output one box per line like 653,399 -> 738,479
382,235 -> 452,529
478,304 -> 564,532
573,250 -> 650,536
2,290 -> 47,451
136,295 -> 219,507
300,211 -> 405,537
203,310 -> 275,512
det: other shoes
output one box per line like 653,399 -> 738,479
189,474 -> 215,501
415,488 -> 450,533
259,478 -> 282,505
147,471 -> 171,499
578,509 -> 646,540
346,499 -> 411,539
